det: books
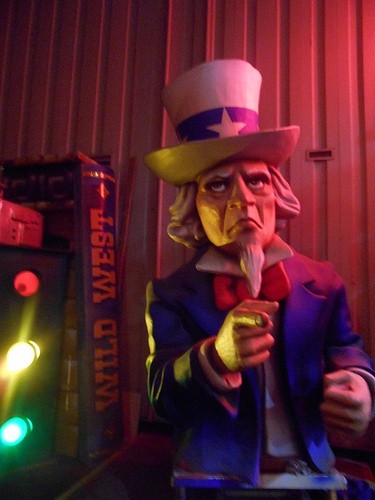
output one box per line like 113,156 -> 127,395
1,159 -> 125,462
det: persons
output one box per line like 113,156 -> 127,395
145,59 -> 374,500
